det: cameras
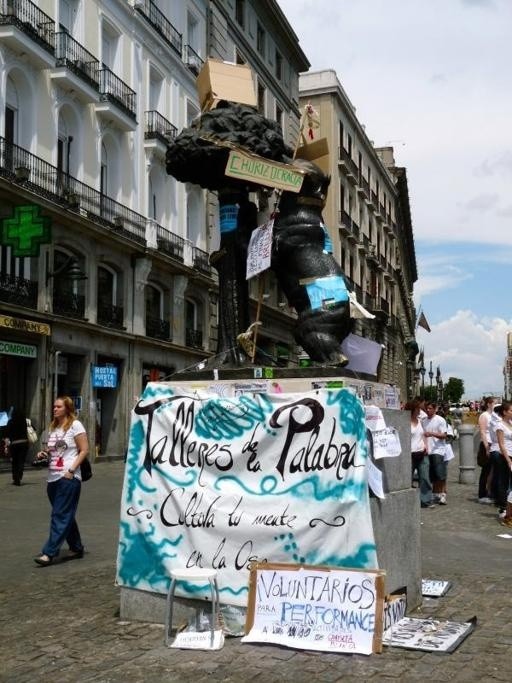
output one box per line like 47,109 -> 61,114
31,459 -> 49,467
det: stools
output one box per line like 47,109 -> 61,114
163,566 -> 226,649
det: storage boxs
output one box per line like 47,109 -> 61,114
195,57 -> 259,113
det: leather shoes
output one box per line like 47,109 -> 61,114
62,552 -> 84,560
33,552 -> 55,566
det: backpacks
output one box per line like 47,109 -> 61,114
80,454 -> 92,481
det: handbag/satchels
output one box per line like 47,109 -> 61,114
26,417 -> 38,444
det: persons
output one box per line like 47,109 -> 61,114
4,406 -> 37,486
401,395 -> 512,524
36,396 -> 91,566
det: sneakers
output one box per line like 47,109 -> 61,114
421,493 -> 447,507
479,495 -> 494,503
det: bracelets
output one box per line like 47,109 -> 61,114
68,468 -> 74,474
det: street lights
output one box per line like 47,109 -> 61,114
427,371 -> 433,399
420,364 -> 426,399
